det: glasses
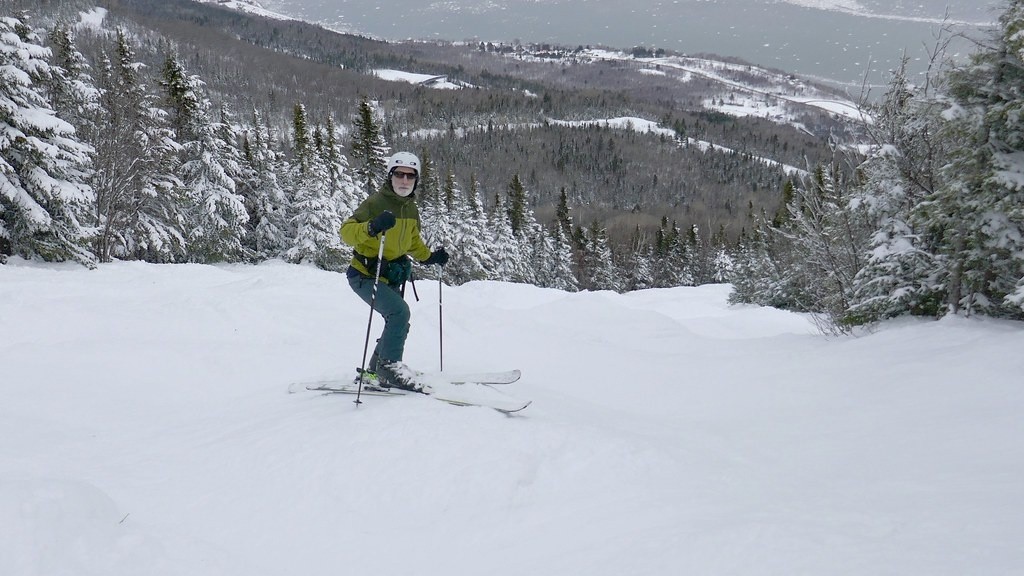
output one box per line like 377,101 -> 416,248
392,171 -> 417,179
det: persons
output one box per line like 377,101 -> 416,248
339,152 -> 449,391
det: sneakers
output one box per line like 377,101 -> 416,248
366,352 -> 378,374
376,360 -> 431,394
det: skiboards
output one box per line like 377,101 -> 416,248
287,369 -> 534,414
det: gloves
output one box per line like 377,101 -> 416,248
371,211 -> 396,235
420,249 -> 450,267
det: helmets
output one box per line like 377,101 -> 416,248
387,152 -> 422,178
